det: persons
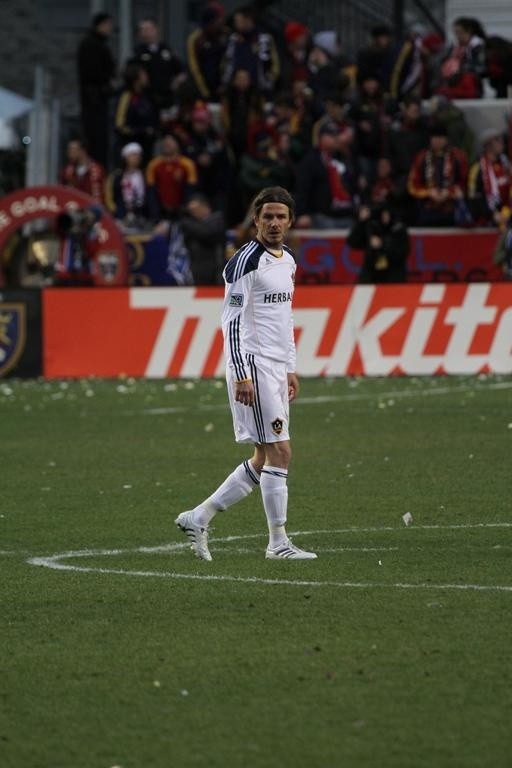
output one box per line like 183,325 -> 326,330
173,185 -> 317,563
59,2 -> 511,281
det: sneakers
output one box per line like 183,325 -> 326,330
173,510 -> 213,562
263,540 -> 317,562
